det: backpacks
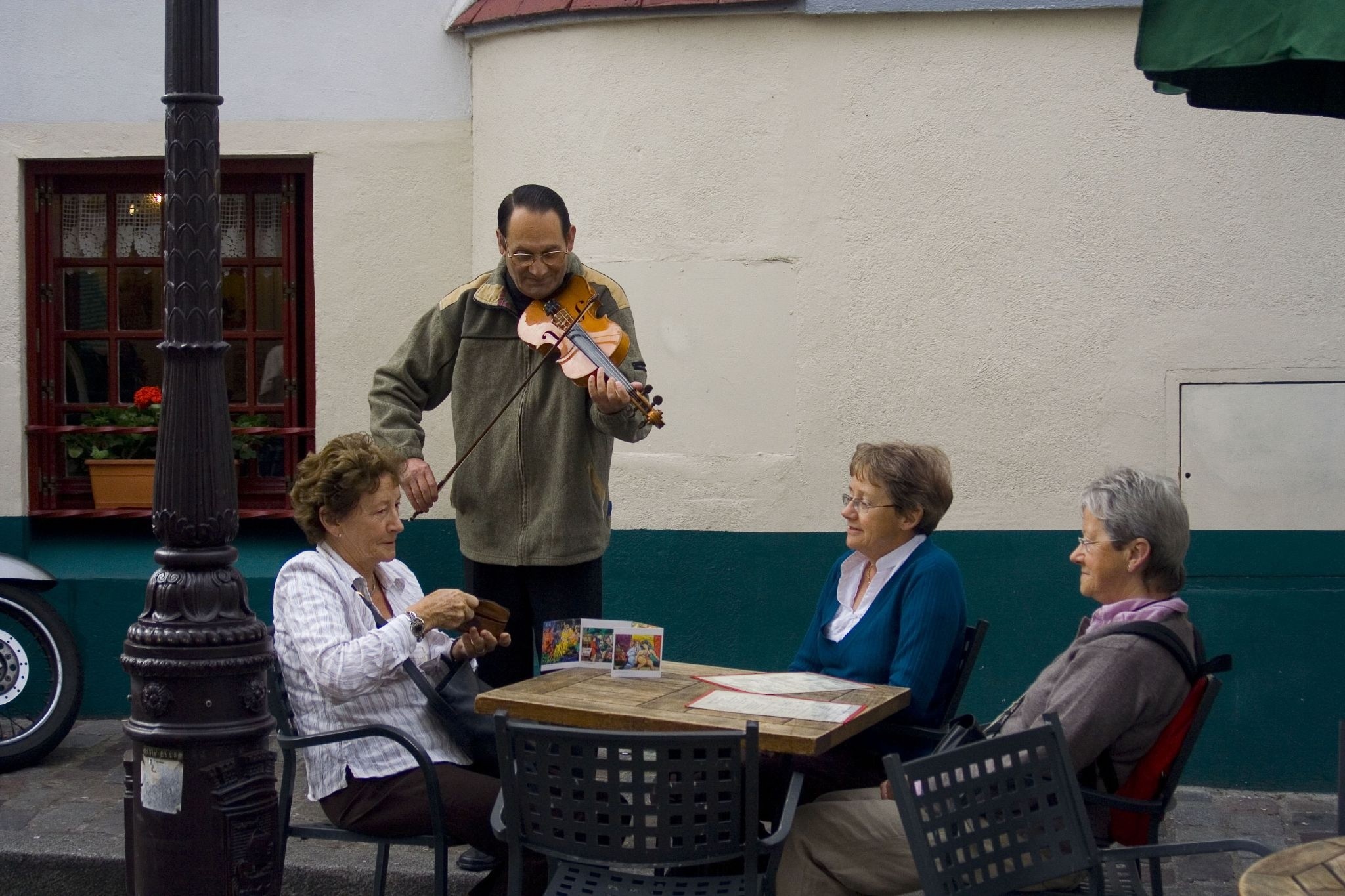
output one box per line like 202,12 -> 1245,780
1103,619 -> 1234,849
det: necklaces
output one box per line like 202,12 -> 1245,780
867,563 -> 873,585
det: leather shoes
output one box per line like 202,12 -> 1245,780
456,847 -> 495,871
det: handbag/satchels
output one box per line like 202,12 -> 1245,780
426,659 -> 515,770
928,714 -> 986,756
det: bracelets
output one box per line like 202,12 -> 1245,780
403,611 -> 425,642
450,636 -> 462,663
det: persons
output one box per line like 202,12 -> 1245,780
272,430 -> 632,896
774,466 -> 1203,896
666,440 -> 966,880
368,184 -> 655,873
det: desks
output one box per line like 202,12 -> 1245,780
473,661 -> 911,760
1237,835 -> 1345,895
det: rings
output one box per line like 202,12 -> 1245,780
476,648 -> 485,654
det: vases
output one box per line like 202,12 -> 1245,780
83,457 -> 241,510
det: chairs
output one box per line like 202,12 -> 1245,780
1081,625 -> 1220,896
494,708 -> 803,896
264,626 -> 471,896
925,619 -> 993,753
881,712 -> 1274,896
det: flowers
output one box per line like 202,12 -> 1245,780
67,387 -> 266,460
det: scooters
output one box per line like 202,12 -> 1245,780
0,548 -> 84,774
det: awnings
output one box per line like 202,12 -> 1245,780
1134,0 -> 1345,119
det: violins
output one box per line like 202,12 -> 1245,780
516,272 -> 666,430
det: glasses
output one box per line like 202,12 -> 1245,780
503,237 -> 568,267
1078,536 -> 1123,545
842,493 -> 903,515
